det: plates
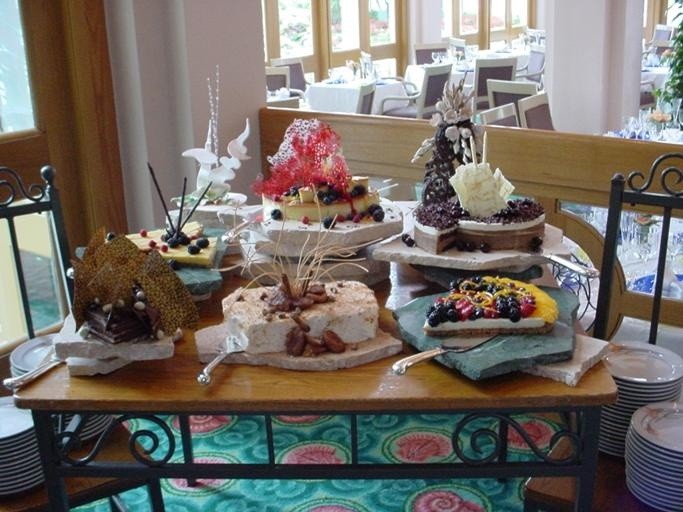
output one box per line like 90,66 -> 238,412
0,396 -> 47,495
10,333 -> 113,446
596,341 -> 682,511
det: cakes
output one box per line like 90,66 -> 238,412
123,157 -> 230,304
240,116 -> 402,284
401,71 -> 547,256
422,275 -> 559,337
54,228 -> 198,377
161,69 -> 264,256
194,202 -> 403,369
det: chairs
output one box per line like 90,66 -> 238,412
521,153 -> 682,511
1,165 -> 165,511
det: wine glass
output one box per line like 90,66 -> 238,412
334,50 -> 380,85
431,45 -> 462,68
518,33 -> 545,51
464,43 -> 481,67
578,97 -> 683,284
